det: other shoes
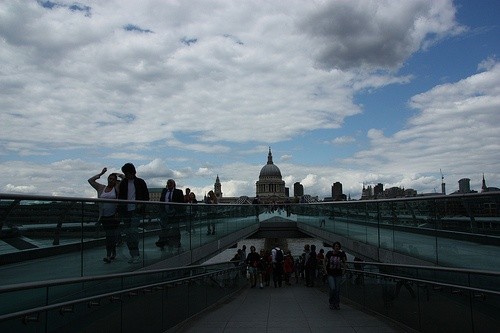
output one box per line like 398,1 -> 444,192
112,257 -> 115,261
128,257 -> 140,264
102,258 -> 111,264
330,304 -> 341,311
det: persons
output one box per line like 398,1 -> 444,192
324,242 -> 346,309
231,242 -> 325,289
185,192 -> 198,234
115,163 -> 149,265
155,178 -> 185,249
206,191 -> 218,235
88,167 -> 125,262
182,188 -> 190,204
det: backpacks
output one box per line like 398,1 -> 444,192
274,248 -> 284,263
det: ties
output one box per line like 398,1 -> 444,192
168,190 -> 173,212
